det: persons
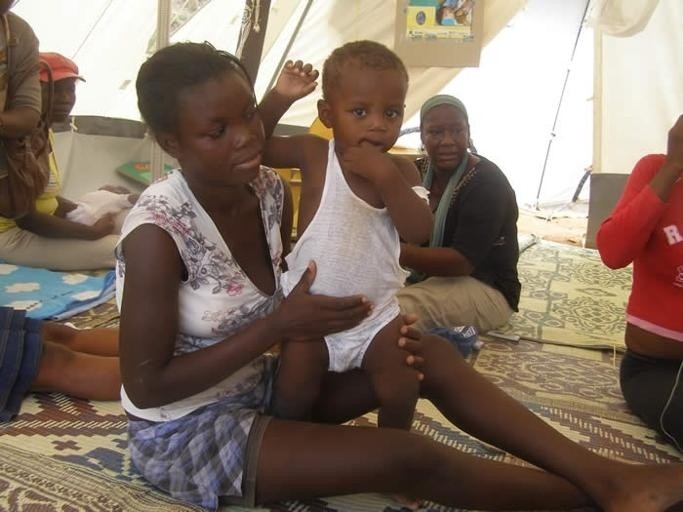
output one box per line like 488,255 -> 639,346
0,51 -> 139,273
0,303 -> 123,421
254,38 -> 434,434
0,0 -> 45,139
593,113 -> 682,457
112,37 -> 683,510
395,94 -> 519,342
47,184 -> 138,230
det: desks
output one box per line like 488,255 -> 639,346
118,160 -> 177,187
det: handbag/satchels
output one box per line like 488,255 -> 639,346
4,59 -> 50,213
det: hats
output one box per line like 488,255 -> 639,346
39,52 -> 87,84
